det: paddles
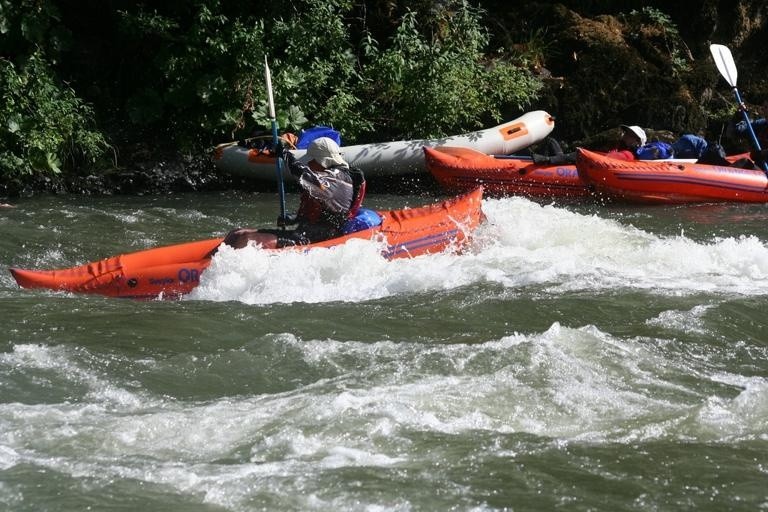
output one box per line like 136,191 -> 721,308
437,146 -> 533,160
264,53 -> 288,222
710,43 -> 761,152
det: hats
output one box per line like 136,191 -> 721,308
620,122 -> 648,148
293,136 -> 350,170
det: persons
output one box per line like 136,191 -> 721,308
201,137 -> 368,261
693,94 -> 768,172
526,123 -> 648,168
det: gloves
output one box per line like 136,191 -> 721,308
263,137 -> 284,158
751,148 -> 768,162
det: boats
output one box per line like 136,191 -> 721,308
421,145 -> 590,197
210,109 -> 556,188
574,145 -> 768,206
8,182 -> 486,305
